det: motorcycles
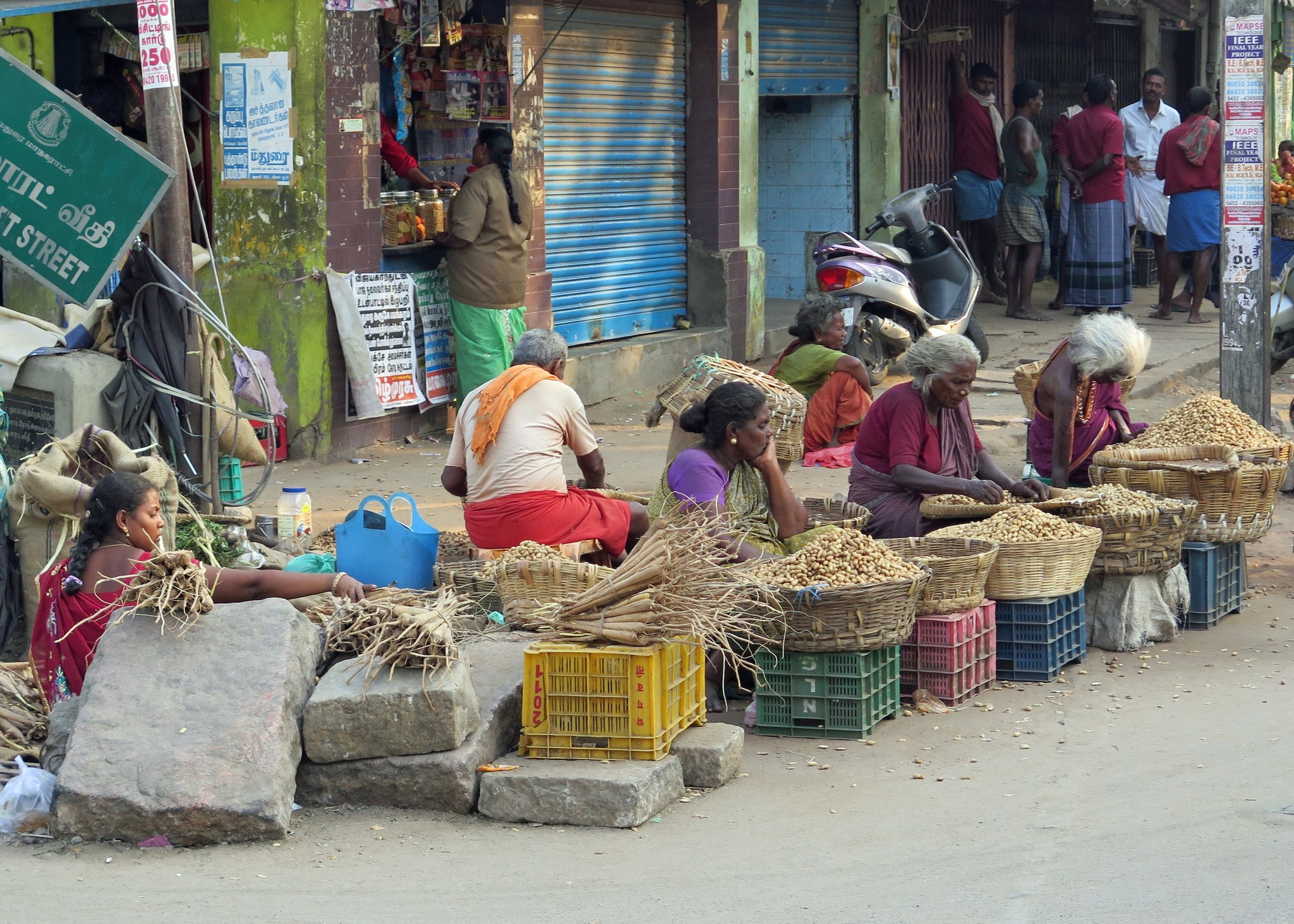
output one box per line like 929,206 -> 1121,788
813,174 -> 989,387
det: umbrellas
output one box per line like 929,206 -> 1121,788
102,238 -> 225,515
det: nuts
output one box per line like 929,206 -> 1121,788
745,527 -> 944,590
946,504 -> 1093,544
308,525 -> 472,557
474,540 -> 577,579
808,649 -> 1237,782
1255,587 -> 1279,620
1042,483 -> 1184,517
1114,395 -> 1288,470
934,489 -> 1082,506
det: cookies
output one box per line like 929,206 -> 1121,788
381,199 -> 425,247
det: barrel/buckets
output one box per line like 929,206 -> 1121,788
255,515 -> 278,538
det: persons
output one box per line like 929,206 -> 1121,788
646,382 -> 843,583
1273,140 -> 1294,167
848,334 -> 1050,540
1150,78 -> 1221,312
431,128 -> 532,418
1148,86 -> 1221,324
441,328 -> 650,568
380,109 -> 461,191
1052,74 -> 1132,317
29,471 -> 377,714
386,0 -> 508,118
1118,67 -> 1181,303
1048,83 -> 1146,309
1029,310 -> 1150,490
951,40 -> 1008,304
768,291 -> 873,460
1000,78 -> 1053,321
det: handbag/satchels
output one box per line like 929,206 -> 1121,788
334,491 -> 440,594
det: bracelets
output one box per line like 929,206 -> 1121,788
332,572 -> 347,597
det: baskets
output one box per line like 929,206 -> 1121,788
871,536 -> 1000,614
565,478 -> 623,499
1012,359 -> 1136,420
735,558 -> 933,652
924,523 -> 1102,600
797,497 -> 871,531
1271,211 -> 1294,240
432,557 -> 504,619
490,563 -> 616,624
1088,452 -> 1290,543
656,355 -> 807,460
1107,439 -> 1294,468
1061,491 -> 1199,577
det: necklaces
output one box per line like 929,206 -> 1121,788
1075,362 -> 1096,425
93,544 -> 130,549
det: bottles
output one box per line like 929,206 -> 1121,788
394,190 -> 416,244
275,427 -> 279,448
438,187 -> 458,232
416,189 -> 443,240
414,192 -> 426,241
380,192 -> 398,248
277,487 -> 312,538
461,166 -> 477,187
255,428 -> 267,440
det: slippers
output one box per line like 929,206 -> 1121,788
1151,301 -> 1191,313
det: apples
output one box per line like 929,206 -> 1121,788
1271,150 -> 1294,205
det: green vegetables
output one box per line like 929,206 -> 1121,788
177,519 -> 248,570
